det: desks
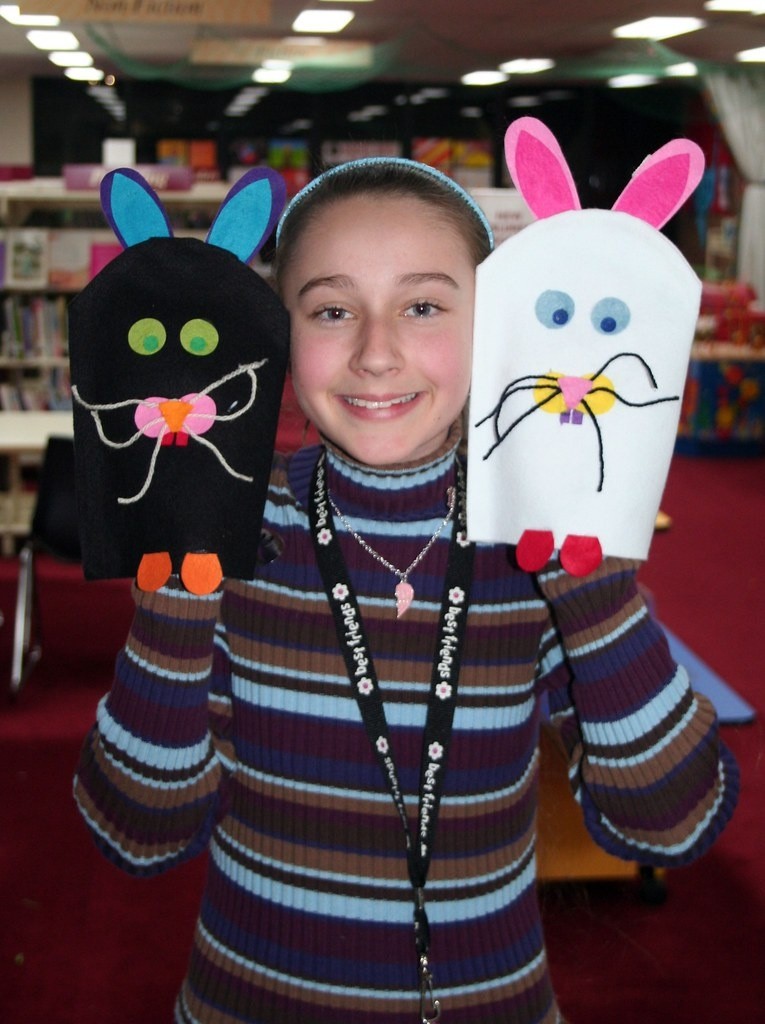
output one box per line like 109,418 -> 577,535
0,408 -> 74,700
688,339 -> 765,461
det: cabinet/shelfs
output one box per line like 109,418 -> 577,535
0,187 -> 274,557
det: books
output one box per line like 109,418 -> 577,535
0,141 -> 495,486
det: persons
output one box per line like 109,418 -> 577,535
71,157 -> 738,1023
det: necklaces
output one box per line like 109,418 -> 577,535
327,488 -> 456,619
